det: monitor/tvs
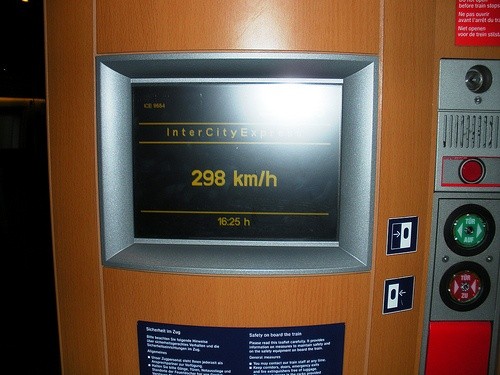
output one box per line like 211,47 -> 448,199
96,51 -> 373,273
